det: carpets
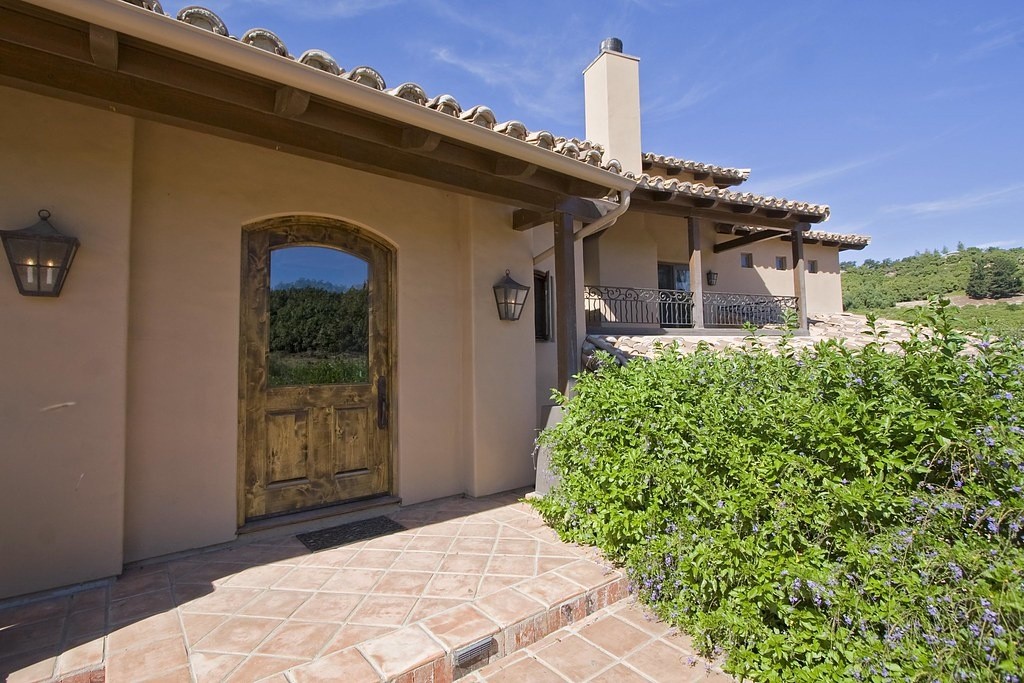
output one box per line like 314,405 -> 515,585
297,515 -> 408,554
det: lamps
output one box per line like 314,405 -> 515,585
492,269 -> 530,321
706,270 -> 718,286
0,209 -> 81,298
